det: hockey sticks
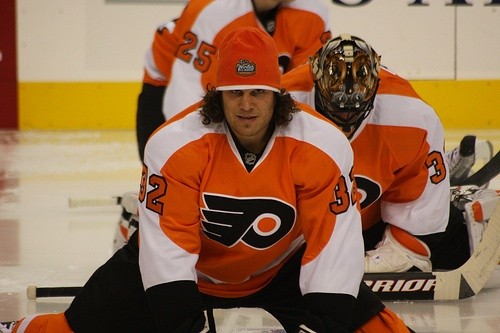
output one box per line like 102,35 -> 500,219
25,200 -> 499,302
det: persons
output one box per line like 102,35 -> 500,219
110,0 -> 331,255
0,25 -> 420,333
280,35 -> 451,272
